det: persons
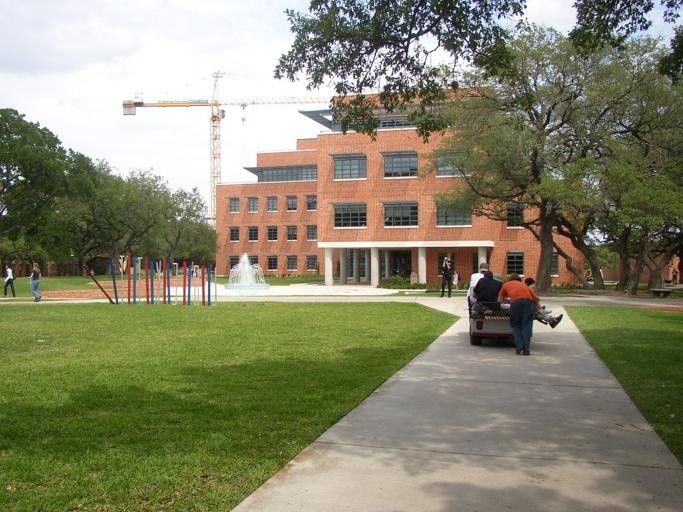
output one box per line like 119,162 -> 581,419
496,274 -> 540,355
2,265 -> 16,297
467,263 -> 489,306
438,253 -> 453,297
471,271 -> 503,313
522,277 -> 563,328
27,262 -> 42,302
671,267 -> 678,287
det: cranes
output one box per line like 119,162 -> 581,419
123,69 -> 330,231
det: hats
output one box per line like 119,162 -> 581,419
525,278 -> 535,285
508,275 -> 521,280
480,263 -> 487,267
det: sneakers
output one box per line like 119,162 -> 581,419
549,314 -> 562,328
